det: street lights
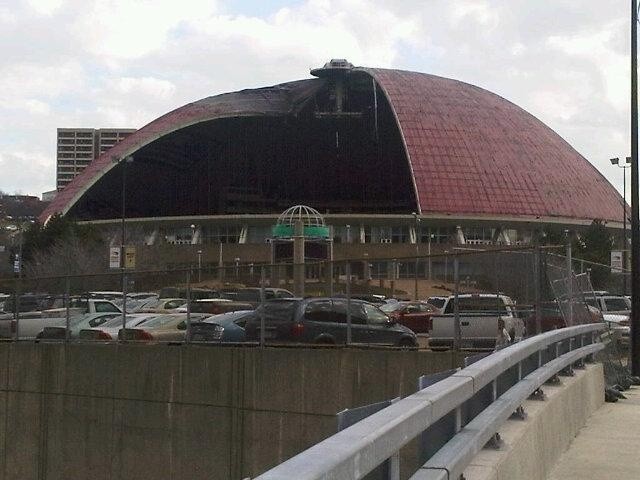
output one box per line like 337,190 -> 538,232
610,156 -> 632,295
411,211 -> 420,243
190,223 -> 197,244
110,153 -> 137,345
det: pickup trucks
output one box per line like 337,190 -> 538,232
425,292 -> 526,351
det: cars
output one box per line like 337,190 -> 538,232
5,224 -> 17,232
515,287 -> 633,353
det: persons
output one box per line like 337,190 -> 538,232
494,319 -> 512,351
